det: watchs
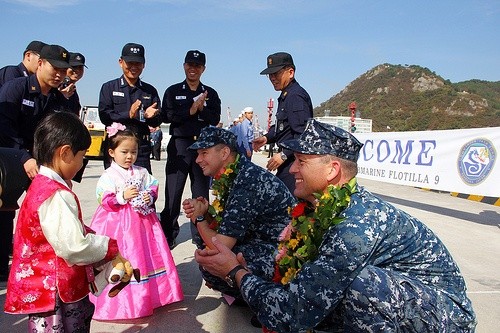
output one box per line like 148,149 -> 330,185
195,216 -> 205,227
280,151 -> 287,161
224,265 -> 247,288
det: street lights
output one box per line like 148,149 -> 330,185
267,98 -> 275,129
349,102 -> 357,133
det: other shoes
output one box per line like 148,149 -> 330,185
222,291 -> 264,328
166,237 -> 175,249
197,241 -> 206,249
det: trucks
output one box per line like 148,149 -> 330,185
79,105 -> 107,158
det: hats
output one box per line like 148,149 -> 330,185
279,117 -> 365,163
40,45 -> 70,69
27,41 -> 48,54
122,43 -> 145,64
259,52 -> 293,74
185,127 -> 238,150
68,53 -> 89,69
219,107 -> 252,129
185,50 -> 205,66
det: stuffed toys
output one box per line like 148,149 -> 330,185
89,252 -> 140,296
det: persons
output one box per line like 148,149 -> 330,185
88,122 -> 183,320
149,126 -> 162,161
228,107 -> 254,163
182,127 -> 295,327
0,41 -> 89,288
252,52 -> 313,194
195,118 -> 478,333
268,124 -> 275,158
6,111 -> 120,333
98,43 -> 164,175
163,50 -> 222,246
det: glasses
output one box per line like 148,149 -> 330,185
69,65 -> 84,70
266,68 -> 291,77
186,62 -> 203,69
